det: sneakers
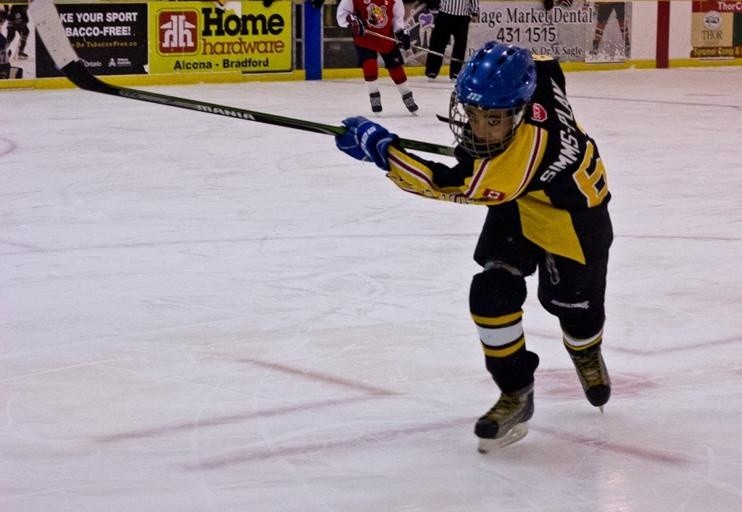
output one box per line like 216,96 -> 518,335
570,348 -> 610,406
475,388 -> 534,438
450,73 -> 458,79
425,70 -> 437,78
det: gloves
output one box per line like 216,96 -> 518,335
335,117 -> 401,171
351,20 -> 365,36
395,28 -> 410,50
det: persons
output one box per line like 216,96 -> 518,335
590,2 -> 630,59
5,5 -> 31,59
0,4 -> 23,79
336,0 -> 419,112
333,38 -> 612,439
425,0 -> 479,79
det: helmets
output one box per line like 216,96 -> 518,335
450,41 -> 536,158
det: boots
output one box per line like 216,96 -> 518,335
370,92 -> 382,111
403,92 -> 418,111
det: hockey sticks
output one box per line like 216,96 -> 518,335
26,0 -> 454,157
365,29 -> 469,63
